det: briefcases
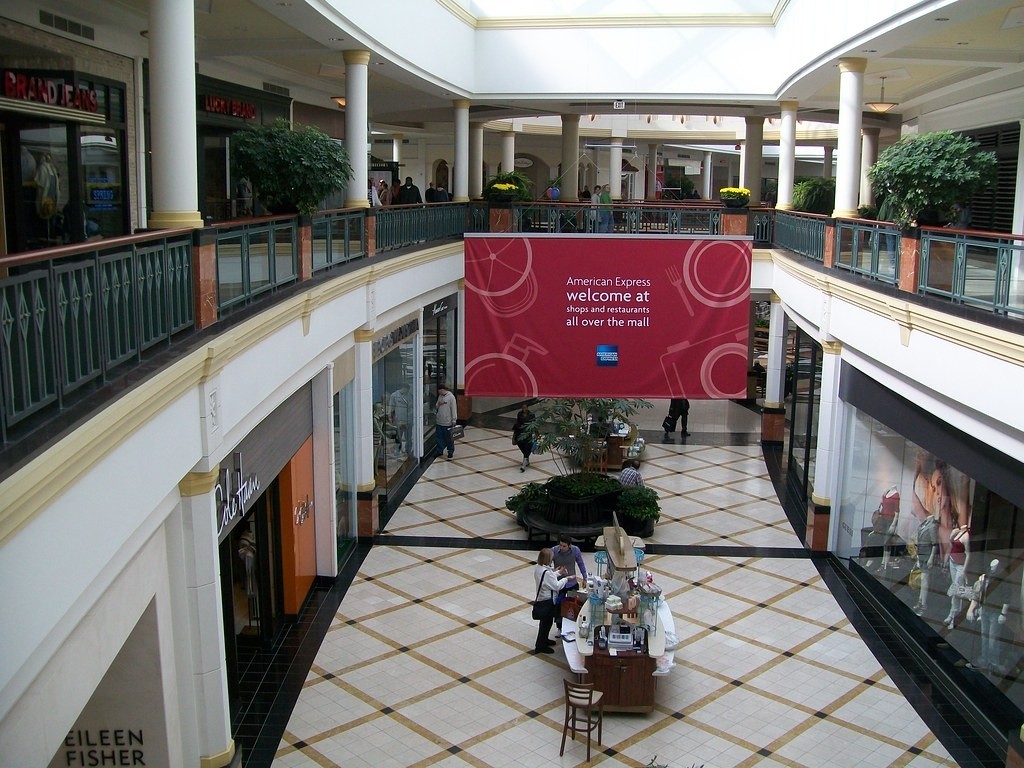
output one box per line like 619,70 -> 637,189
448,422 -> 465,442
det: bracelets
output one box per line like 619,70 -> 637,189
1001,613 -> 1007,616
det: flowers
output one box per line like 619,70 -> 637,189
490,184 -> 519,195
719,187 -> 751,199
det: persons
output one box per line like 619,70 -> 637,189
550,536 -> 588,638
752,360 -> 766,372
368,177 -> 450,211
943,526 -> 970,630
579,184 -> 616,233
915,515 -> 938,610
389,383 -> 412,455
686,190 -> 700,199
373,403 -> 398,478
911,451 -> 970,558
878,186 -> 905,268
19,144 -> 59,221
435,384 -> 458,461
618,460 -> 644,486
516,403 -> 541,472
937,559 -> 1011,671
879,484 -> 900,572
534,548 -> 577,654
664,398 -> 690,439
238,530 -> 256,595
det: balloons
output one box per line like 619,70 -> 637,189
547,187 -> 560,199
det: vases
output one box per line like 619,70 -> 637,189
495,193 -> 514,202
720,197 -> 749,208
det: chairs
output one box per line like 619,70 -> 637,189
559,679 -> 603,763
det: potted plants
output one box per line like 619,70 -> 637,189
505,397 -> 662,548
857,130 -> 999,294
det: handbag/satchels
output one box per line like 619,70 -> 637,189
662,413 -> 675,432
908,561 -> 922,593
873,504 -> 884,534
561,595 -> 584,621
532,599 -> 558,619
511,432 -> 517,445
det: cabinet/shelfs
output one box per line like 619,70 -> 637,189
585,625 -> 657,715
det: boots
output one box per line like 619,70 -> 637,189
520,457 -> 529,472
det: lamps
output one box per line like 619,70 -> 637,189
864,75 -> 900,113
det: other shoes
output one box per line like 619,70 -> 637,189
546,639 -> 557,647
535,646 -> 554,654
554,630 -> 561,637
433,452 -> 443,457
448,452 -> 452,459
664,434 -> 669,441
913,600 -> 928,615
681,431 -> 691,437
876,564 -> 884,573
